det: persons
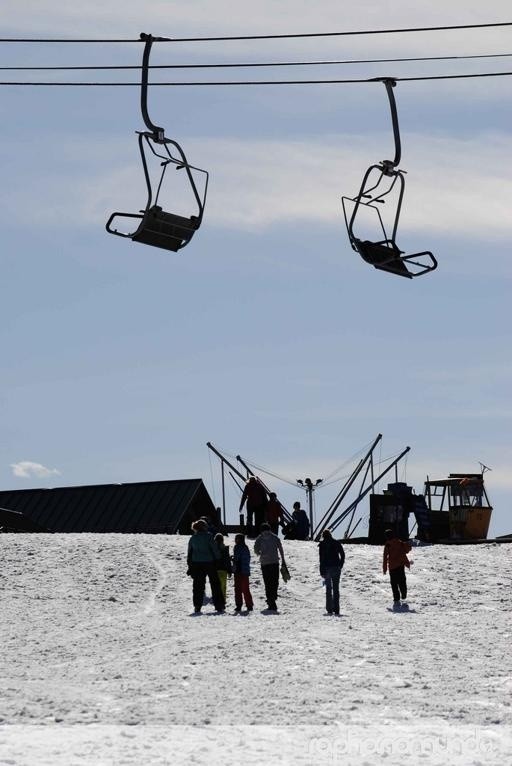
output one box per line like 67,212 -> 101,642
231,535 -> 253,610
187,519 -> 232,613
383,530 -> 410,601
318,530 -> 345,614
254,523 -> 286,609
284,502 -> 309,540
239,477 -> 284,538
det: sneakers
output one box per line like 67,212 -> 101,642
394,593 -> 407,601
192,605 -> 341,618
